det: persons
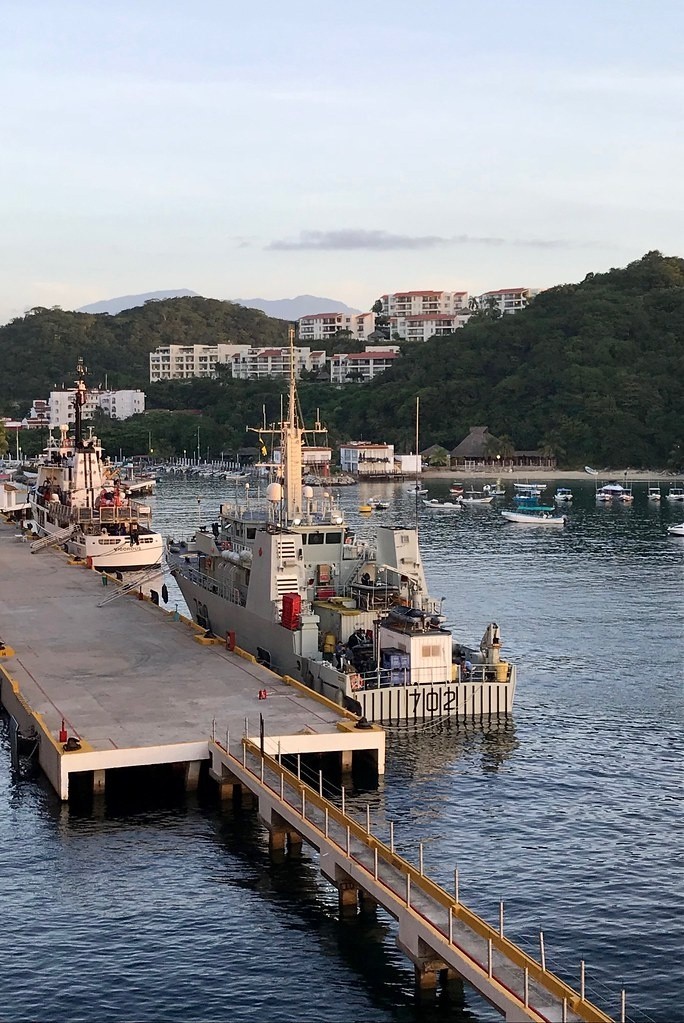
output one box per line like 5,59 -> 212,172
461,660 -> 473,679
336,641 -> 344,669
345,646 -> 354,664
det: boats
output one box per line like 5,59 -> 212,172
667,521 -> 684,536
420,498 -> 462,508
665,488 -> 684,501
554,487 -> 574,501
144,464 -> 253,480
165,327 -> 517,721
618,489 -> 635,501
455,495 -> 495,507
488,474 -> 506,496
405,488 -> 429,495
448,480 -> 465,494
21,356 -> 164,568
647,488 -> 662,500
596,492 -> 613,501
358,498 -> 392,513
517,502 -> 557,513
500,510 -> 567,524
512,479 -> 549,502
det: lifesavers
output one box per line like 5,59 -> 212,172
351,676 -> 363,689
221,542 -> 230,550
